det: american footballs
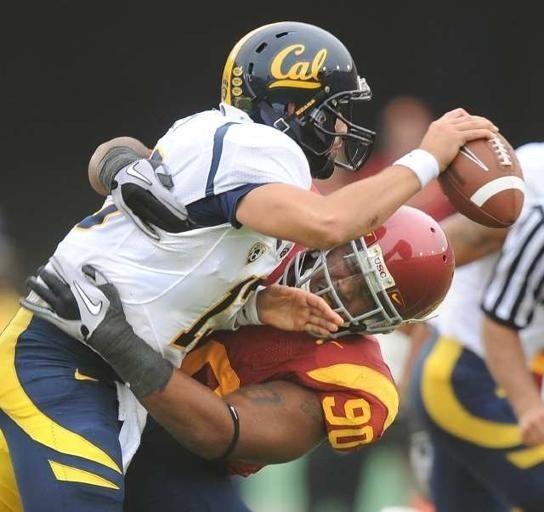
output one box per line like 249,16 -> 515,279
437,132 -> 525,228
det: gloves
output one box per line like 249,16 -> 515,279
96,146 -> 189,241
18,255 -> 174,398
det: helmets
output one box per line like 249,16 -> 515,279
353,205 -> 456,325
220,20 -> 362,137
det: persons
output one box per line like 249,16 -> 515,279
0,21 -> 544,512
